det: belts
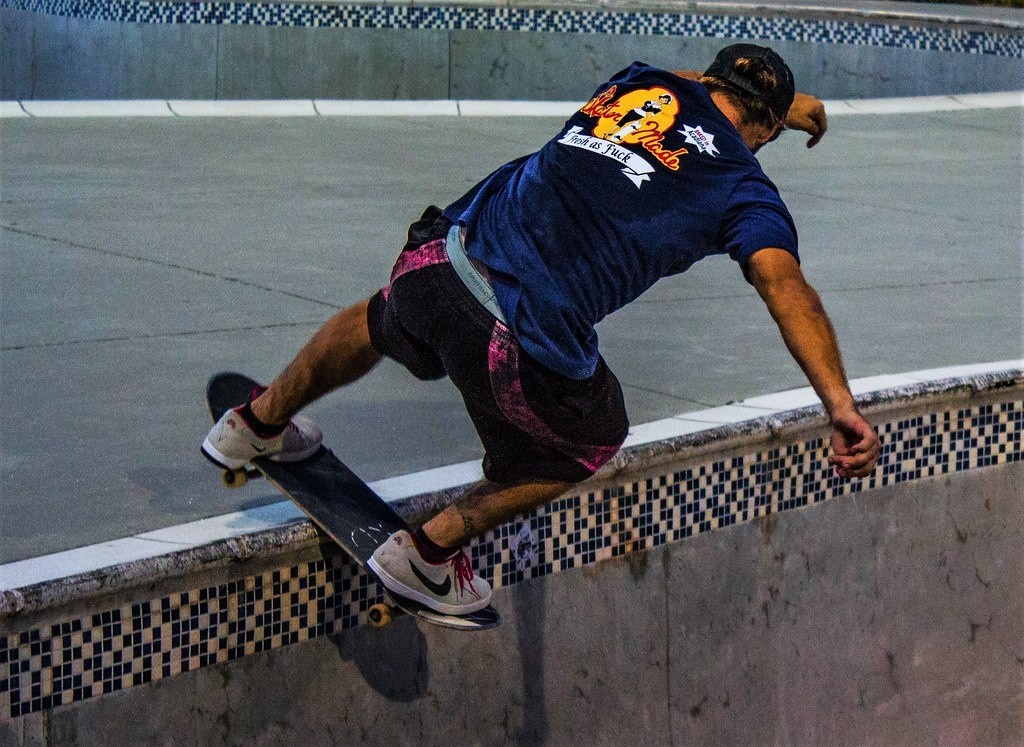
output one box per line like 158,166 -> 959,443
445,223 -> 509,325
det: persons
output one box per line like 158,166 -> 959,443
203,42 -> 883,616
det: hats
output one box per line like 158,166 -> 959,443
703,43 -> 795,120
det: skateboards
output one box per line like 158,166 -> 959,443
204,373 -> 501,635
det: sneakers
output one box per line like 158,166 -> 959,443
366,528 -> 492,615
200,387 -> 323,470
